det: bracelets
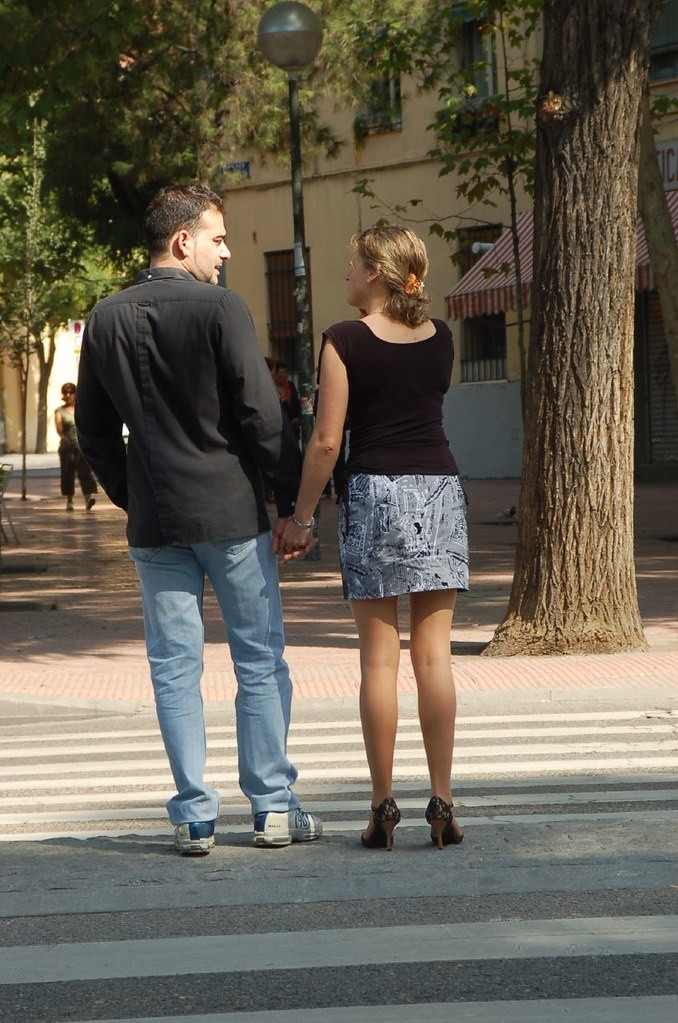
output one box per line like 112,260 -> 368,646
292,514 -> 315,528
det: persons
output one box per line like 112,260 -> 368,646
263,356 -> 303,502
74,182 -> 323,854
279,224 -> 471,852
54,382 -> 98,510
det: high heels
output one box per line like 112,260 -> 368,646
425,798 -> 464,849
361,798 -> 401,849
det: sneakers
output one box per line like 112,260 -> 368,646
173,817 -> 216,853
252,809 -> 322,846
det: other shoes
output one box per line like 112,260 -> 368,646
336,497 -> 340,505
266,496 -> 275,503
67,503 -> 72,511
87,498 -> 95,509
319,493 -> 331,499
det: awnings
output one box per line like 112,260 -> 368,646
445,187 -> 678,318
